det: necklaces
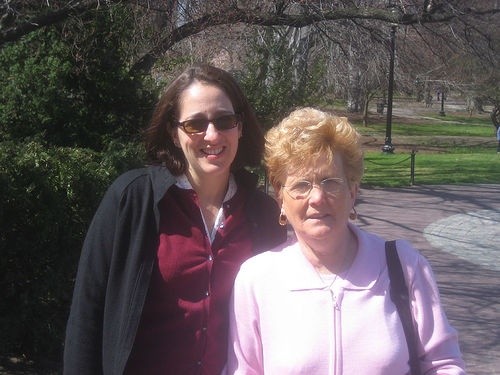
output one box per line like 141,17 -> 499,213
329,232 -> 354,288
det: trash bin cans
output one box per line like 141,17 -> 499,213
376,103 -> 384,114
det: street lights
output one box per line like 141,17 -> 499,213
382,6 -> 399,153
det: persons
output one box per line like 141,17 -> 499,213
221,107 -> 466,375
62,64 -> 287,375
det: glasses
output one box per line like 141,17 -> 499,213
171,111 -> 239,133
278,178 -> 355,200
